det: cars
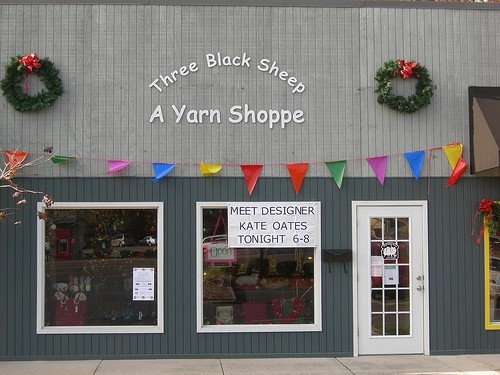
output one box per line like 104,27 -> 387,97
139,236 -> 156,247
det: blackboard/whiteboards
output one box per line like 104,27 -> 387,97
203,241 -> 238,263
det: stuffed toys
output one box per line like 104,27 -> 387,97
53,282 -> 87,301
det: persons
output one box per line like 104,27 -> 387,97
68,273 -> 94,292
264,255 -> 277,273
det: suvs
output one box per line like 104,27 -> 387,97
110,233 -> 135,247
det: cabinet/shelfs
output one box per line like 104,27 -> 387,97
235,289 -> 314,324
